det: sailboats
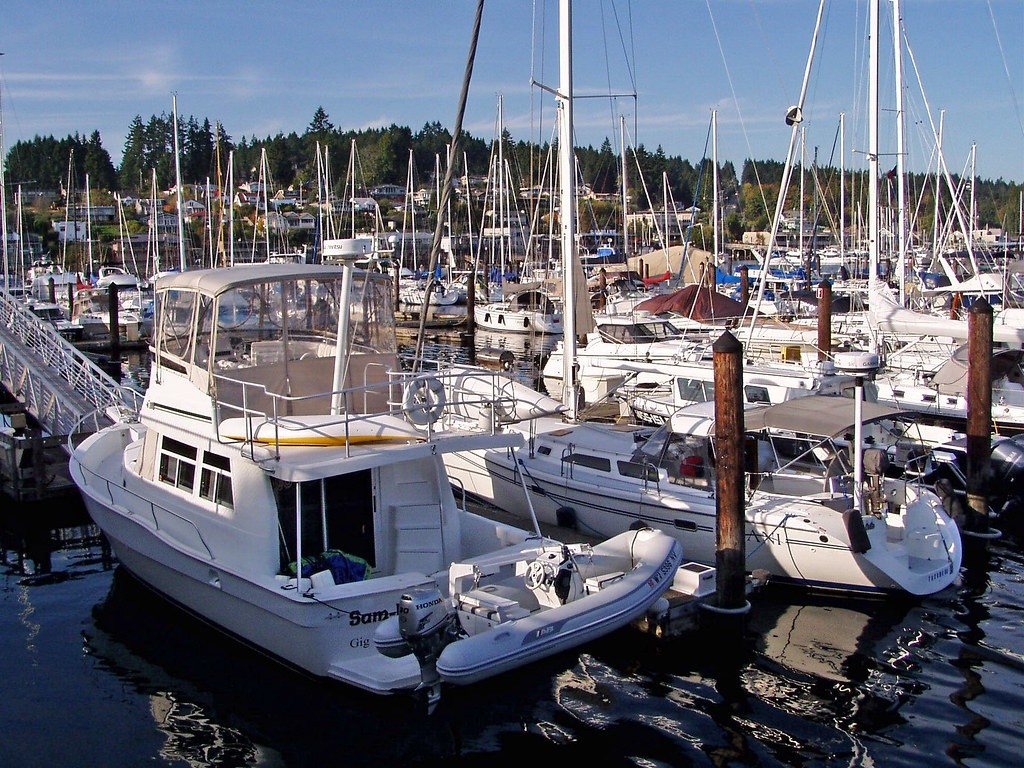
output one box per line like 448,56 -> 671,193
0,0 -> 1024,696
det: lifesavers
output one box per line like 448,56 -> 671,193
399,372 -> 449,428
